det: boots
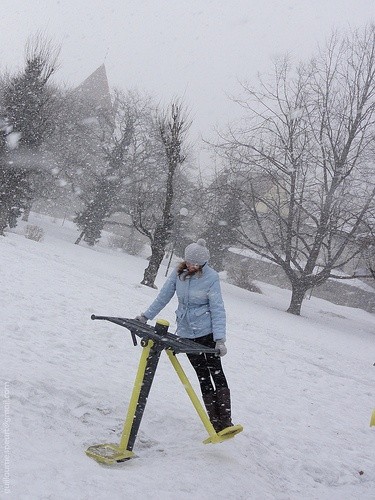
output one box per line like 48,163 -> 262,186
215,389 -> 233,429
202,391 -> 221,432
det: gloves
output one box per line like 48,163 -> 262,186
215,340 -> 227,357
135,313 -> 148,323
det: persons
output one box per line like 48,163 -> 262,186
135,238 -> 235,434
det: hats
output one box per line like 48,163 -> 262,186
185,238 -> 210,265
